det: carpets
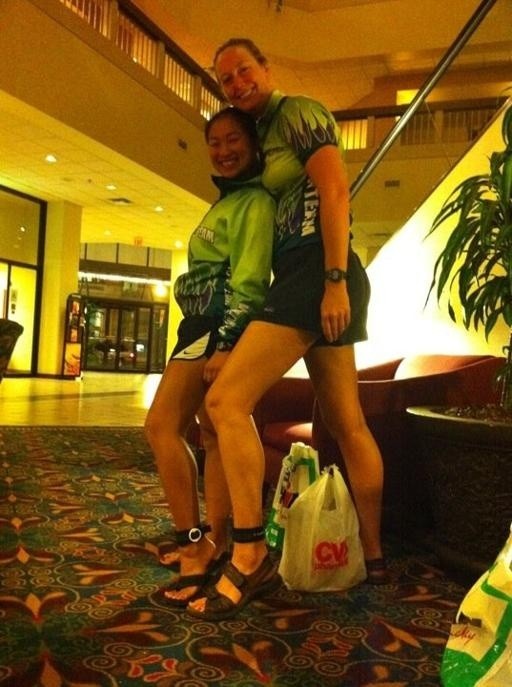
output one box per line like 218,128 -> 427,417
0,424 -> 496,687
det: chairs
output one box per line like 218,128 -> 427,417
252,353 -> 507,536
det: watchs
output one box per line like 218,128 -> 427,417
323,266 -> 351,283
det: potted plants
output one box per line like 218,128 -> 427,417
403,97 -> 512,558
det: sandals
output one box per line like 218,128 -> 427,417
362,558 -> 392,585
186,557 -> 283,621
159,524 -> 229,606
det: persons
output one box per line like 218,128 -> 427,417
184,38 -> 391,624
142,106 -> 277,609
